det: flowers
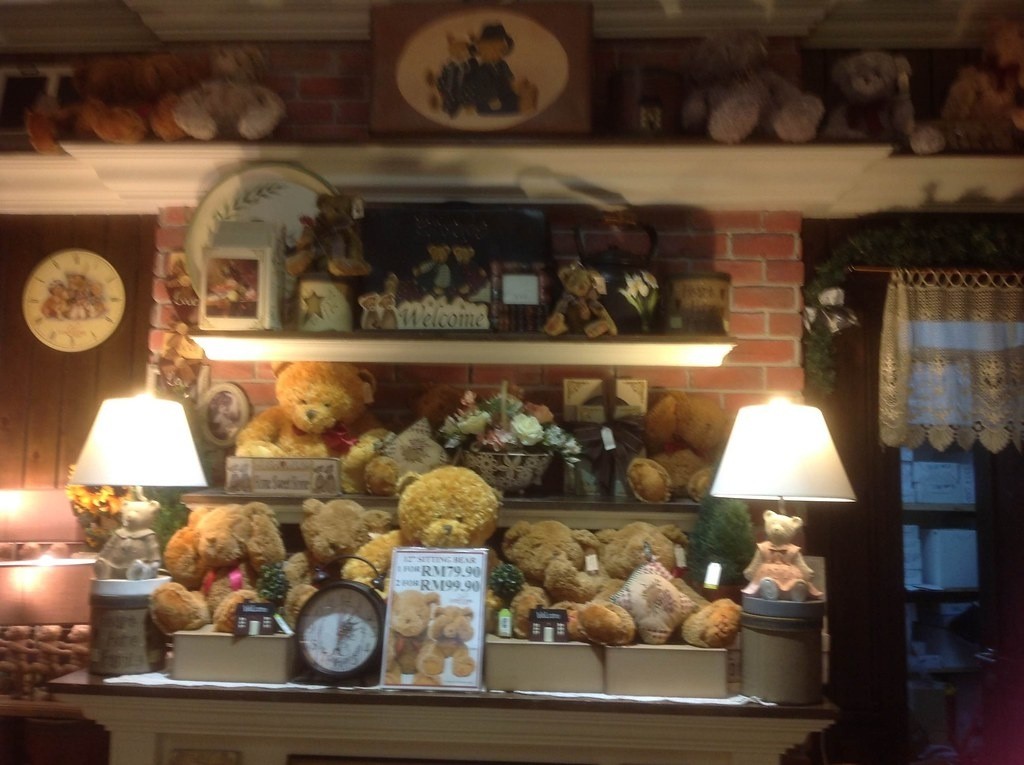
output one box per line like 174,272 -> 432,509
438,384 -> 583,468
65,464 -> 135,553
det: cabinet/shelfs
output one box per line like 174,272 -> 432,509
178,330 -> 738,534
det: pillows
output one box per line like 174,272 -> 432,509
610,563 -> 699,645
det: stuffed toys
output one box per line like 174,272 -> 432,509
23,42 -> 287,151
634,0 -> 1024,158
0,193 -> 823,698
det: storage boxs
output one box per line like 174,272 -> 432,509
368,0 -> 594,138
484,634 -> 605,693
171,631 -> 296,684
561,376 -> 648,498
604,643 -> 728,699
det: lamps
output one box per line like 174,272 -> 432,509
69,389 -> 208,596
709,399 -> 859,619
0,554 -> 94,628
0,488 -> 86,545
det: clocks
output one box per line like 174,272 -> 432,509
22,249 -> 126,354
295,553 -> 387,688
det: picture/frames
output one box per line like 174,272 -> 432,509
200,246 -> 268,330
200,383 -> 251,449
